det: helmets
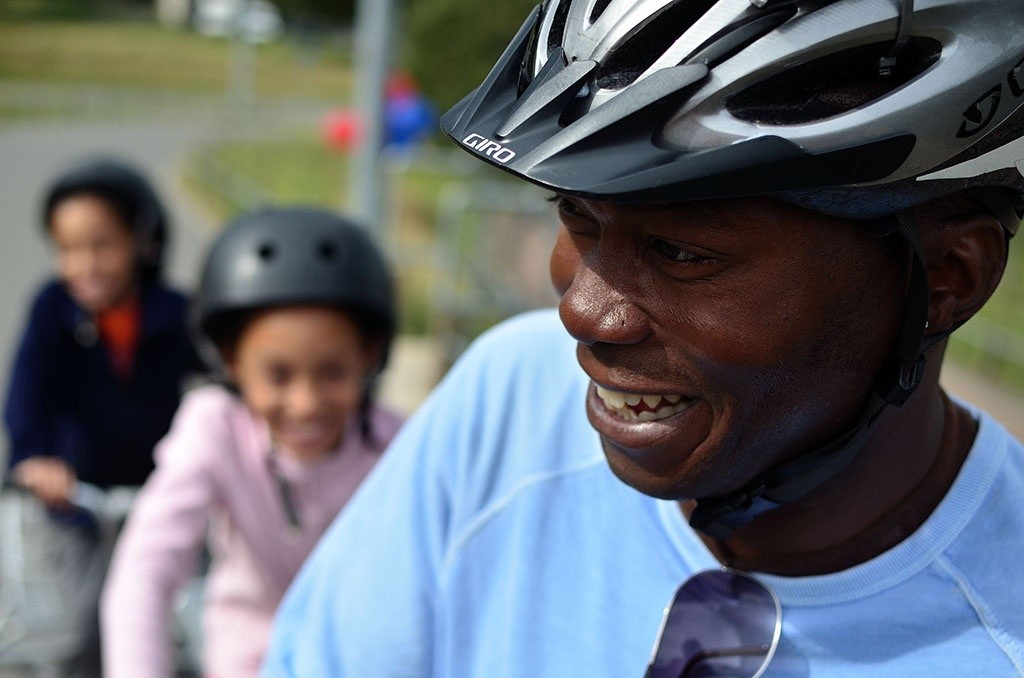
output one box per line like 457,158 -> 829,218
45,155 -> 167,291
438,2 -> 1022,226
188,203 -> 396,372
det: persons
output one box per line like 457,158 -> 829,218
97,209 -> 405,678
0,160 -> 214,678
260,0 -> 1024,678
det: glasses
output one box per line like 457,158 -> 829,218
644,566 -> 781,677
